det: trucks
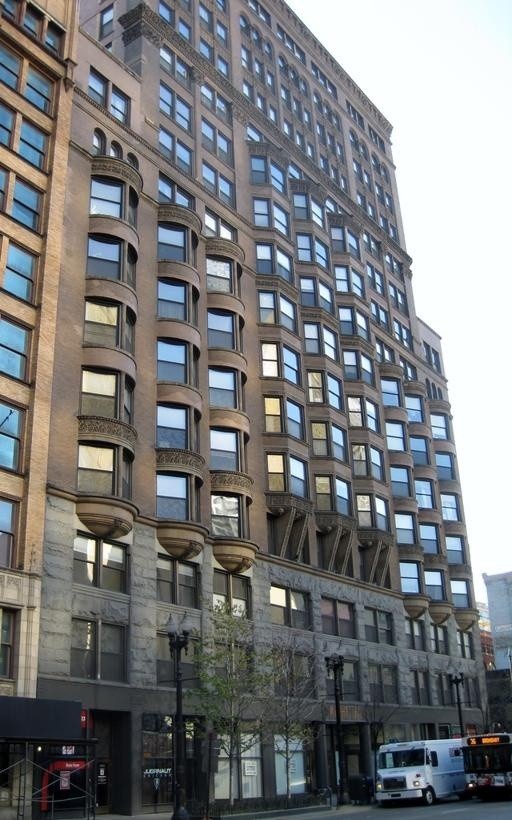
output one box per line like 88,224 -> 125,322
375,737 -> 467,806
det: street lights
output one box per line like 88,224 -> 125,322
322,639 -> 346,809
165,611 -> 194,820
446,658 -> 469,737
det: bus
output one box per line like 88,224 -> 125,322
461,733 -> 511,800
